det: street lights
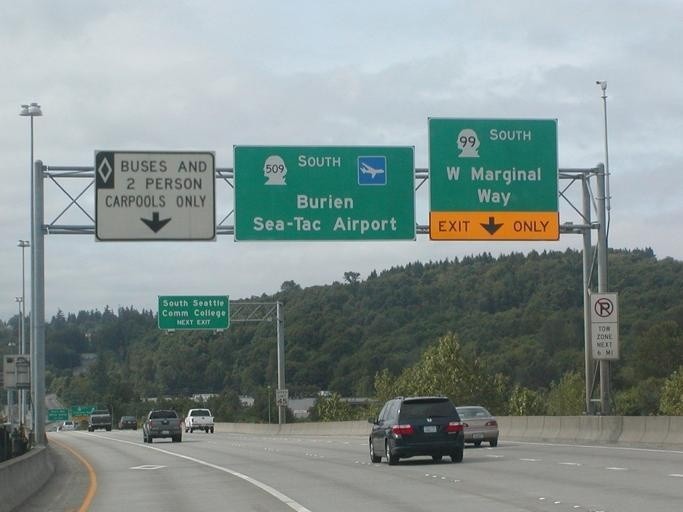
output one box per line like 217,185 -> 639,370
588,80 -> 616,413
7,342 -> 16,422
11,295 -> 22,354
96,151 -> 216,243
20,103 -> 43,433
17,239 -> 30,426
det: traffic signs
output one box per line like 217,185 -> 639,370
429,119 -> 558,241
158,293 -> 228,330
235,144 -> 417,240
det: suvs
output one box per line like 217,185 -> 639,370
367,393 -> 466,465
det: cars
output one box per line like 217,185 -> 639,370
46,407 -> 216,443
453,406 -> 499,452
